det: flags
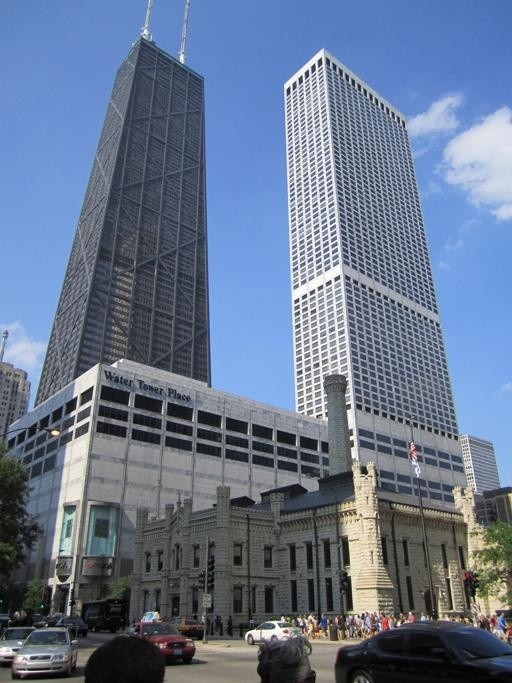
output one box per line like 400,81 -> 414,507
408,441 -> 421,478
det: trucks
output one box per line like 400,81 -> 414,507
81,599 -> 130,633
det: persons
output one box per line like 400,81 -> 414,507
205,615 -> 233,636
84,635 -> 165,683
257,635 -> 316,682
280,611 -> 512,641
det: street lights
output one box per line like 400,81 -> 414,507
0,426 -> 61,438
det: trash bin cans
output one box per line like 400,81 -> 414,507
329,625 -> 338,640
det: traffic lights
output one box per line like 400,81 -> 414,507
207,555 -> 215,590
464,571 -> 476,596
199,571 -> 205,592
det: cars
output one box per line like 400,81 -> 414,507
166,616 -> 204,639
129,620 -> 196,664
1,625 -> 37,664
1,611 -> 88,637
332,619 -> 510,683
245,620 -> 303,646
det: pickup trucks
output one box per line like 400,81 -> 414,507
12,626 -> 80,679
